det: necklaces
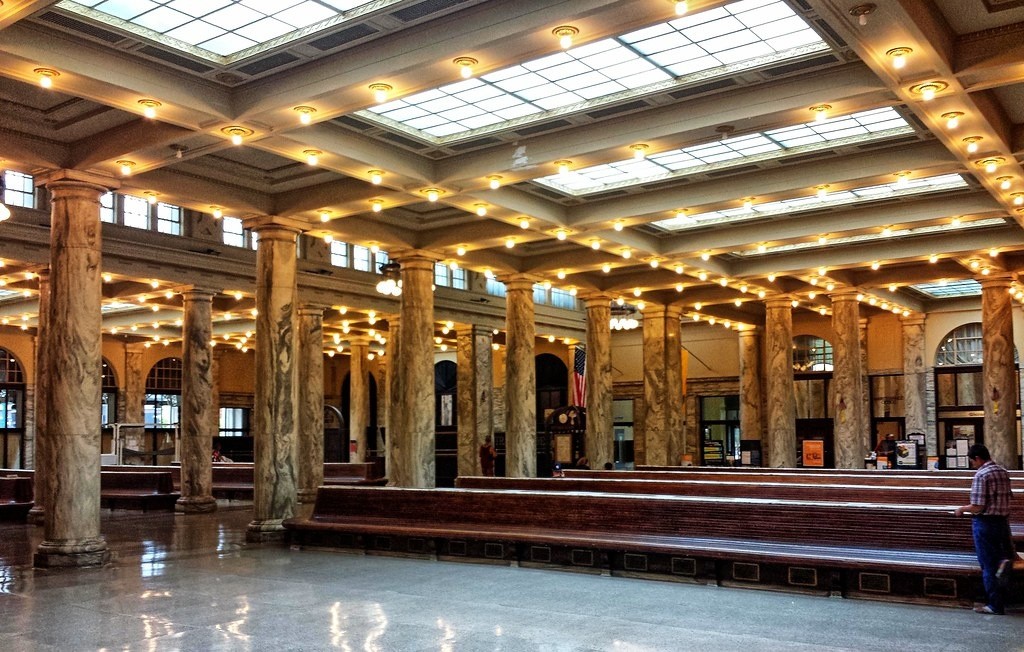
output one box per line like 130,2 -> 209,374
552,463 -> 565,478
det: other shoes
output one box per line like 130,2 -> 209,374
973,606 -> 1004,615
996,559 -> 1012,579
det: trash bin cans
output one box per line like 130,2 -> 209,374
681,455 -> 693,466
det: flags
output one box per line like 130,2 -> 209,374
572,347 -> 586,408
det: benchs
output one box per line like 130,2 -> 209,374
1,462 -> 1023,611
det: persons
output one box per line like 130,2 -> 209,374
576,457 -> 591,470
212,436 -> 233,463
604,462 -> 613,470
874,433 -> 898,469
954,444 -> 1017,614
479,435 -> 497,477
